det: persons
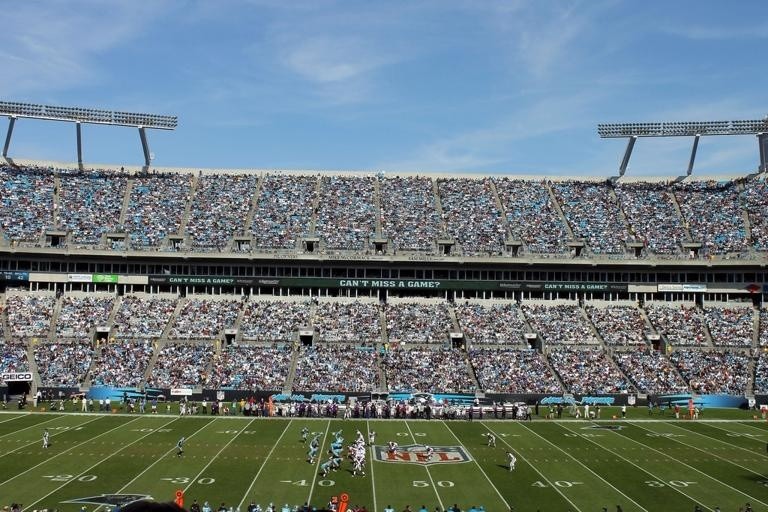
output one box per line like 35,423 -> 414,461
485,431 -> 497,447
1,495 -> 487,512
387,440 -> 400,456
423,444 -> 434,461
744,501 -> 754,512
504,450 -> 517,472
297,426 -> 379,479
41,427 -> 50,449
175,436 -> 185,458
2,161 -> 767,420
